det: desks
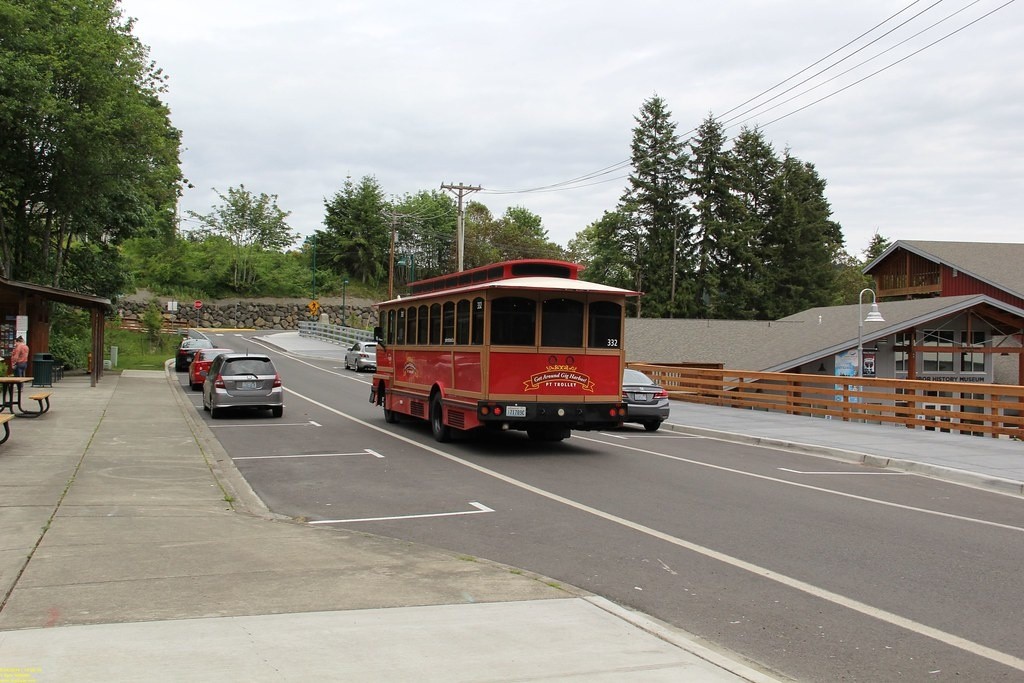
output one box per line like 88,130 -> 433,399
0,376 -> 34,412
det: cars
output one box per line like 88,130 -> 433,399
344,341 -> 378,373
172,338 -> 217,371
187,347 -> 236,391
199,351 -> 284,418
622,366 -> 671,432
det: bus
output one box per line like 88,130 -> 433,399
368,257 -> 645,443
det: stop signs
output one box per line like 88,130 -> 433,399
194,300 -> 203,310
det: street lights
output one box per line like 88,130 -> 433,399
304,233 -> 315,321
858,288 -> 886,423
397,253 -> 413,295
341,278 -> 350,327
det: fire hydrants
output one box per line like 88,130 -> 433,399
85,351 -> 92,374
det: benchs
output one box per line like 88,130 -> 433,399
0,413 -> 15,425
52,361 -> 65,383
29,392 -> 53,400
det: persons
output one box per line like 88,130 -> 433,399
11,336 -> 29,392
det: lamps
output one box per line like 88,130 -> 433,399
818,363 -> 826,371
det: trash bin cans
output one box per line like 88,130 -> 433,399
30,353 -> 56,388
